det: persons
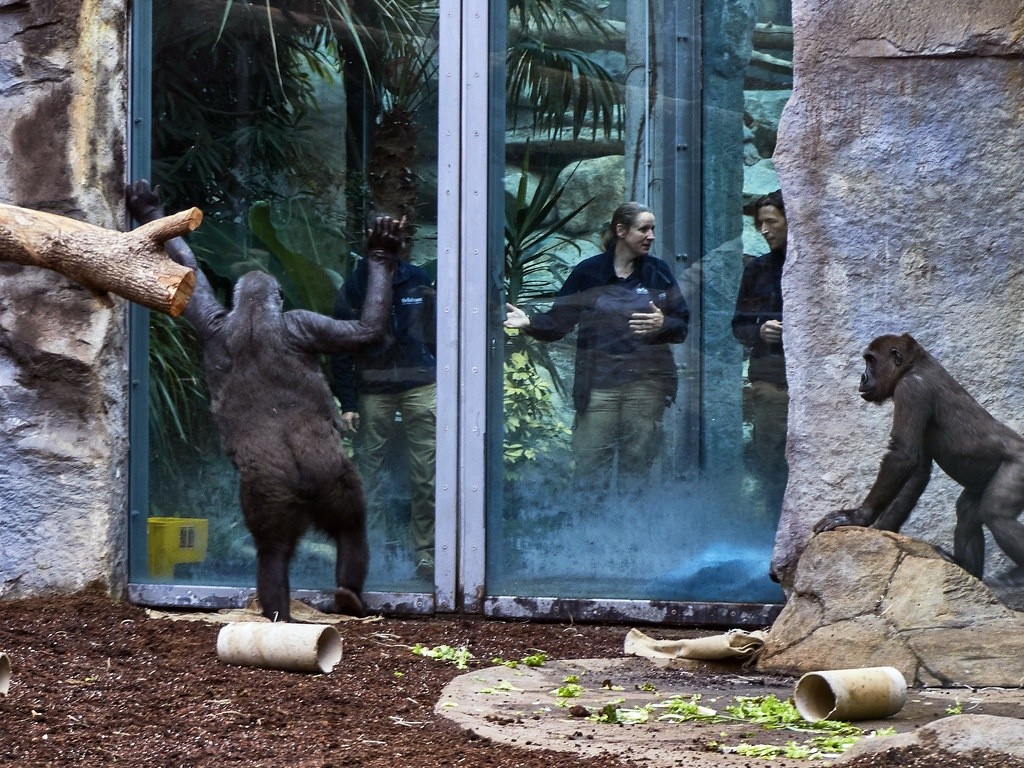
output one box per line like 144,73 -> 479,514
732,189 -> 789,488
503,202 -> 690,500
